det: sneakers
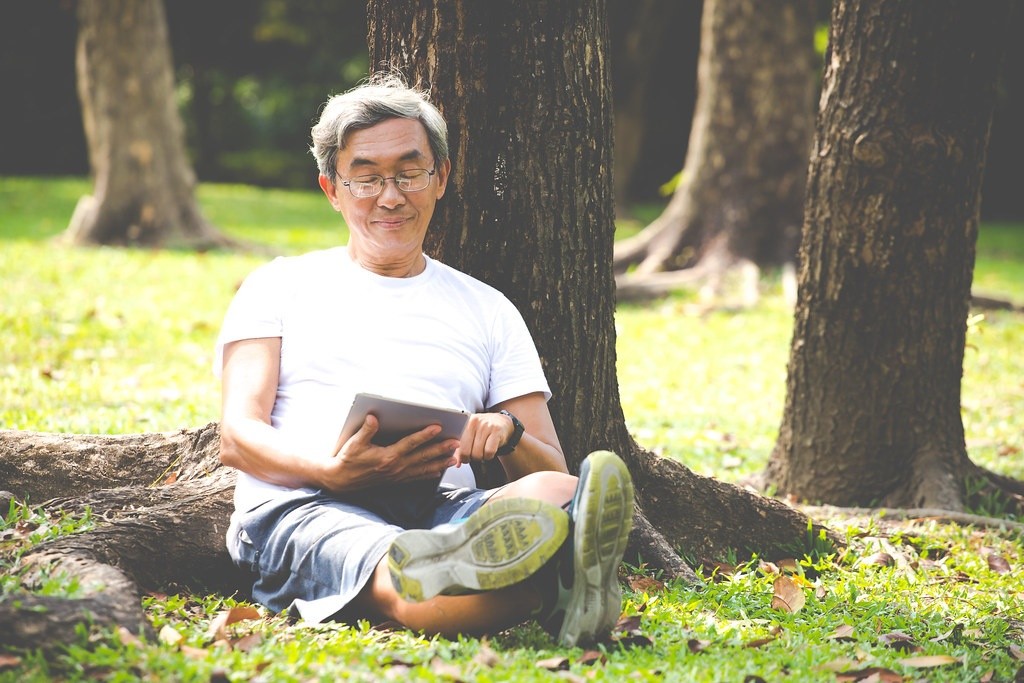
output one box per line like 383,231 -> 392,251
388,497 -> 572,603
531,450 -> 633,649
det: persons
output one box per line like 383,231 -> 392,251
216,70 -> 636,651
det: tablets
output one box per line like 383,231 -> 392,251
319,394 -> 472,515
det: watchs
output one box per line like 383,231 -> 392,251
497,410 -> 526,456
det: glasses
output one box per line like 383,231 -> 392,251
332,158 -> 437,198
